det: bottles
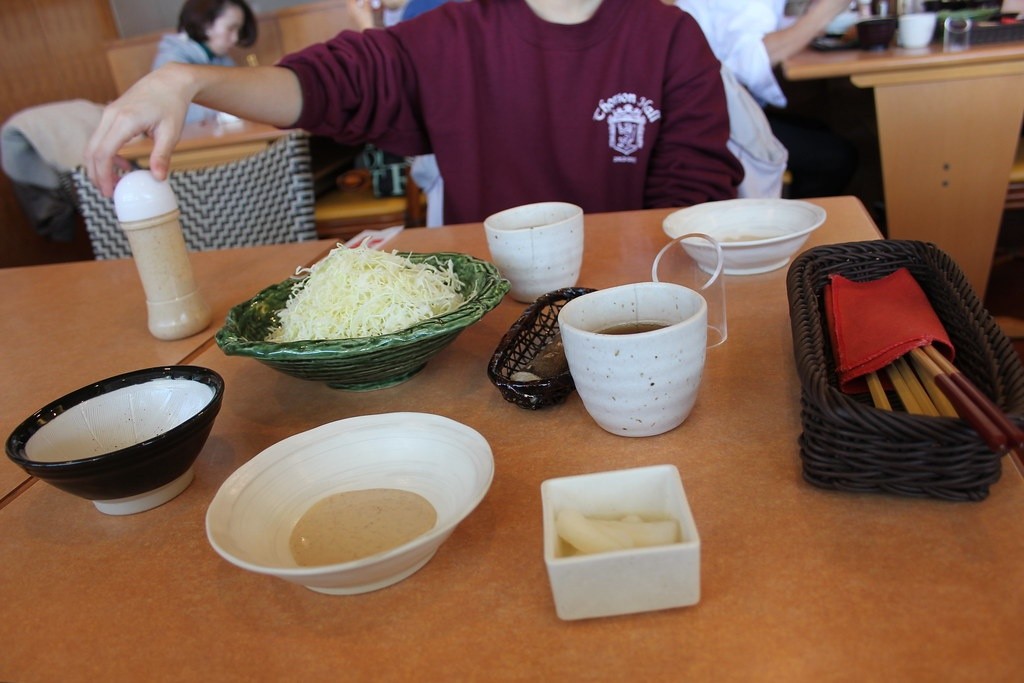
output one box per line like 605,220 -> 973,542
113,169 -> 211,341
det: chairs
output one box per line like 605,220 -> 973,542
71,131 -> 317,260
314,166 -> 428,240
0,99 -> 108,245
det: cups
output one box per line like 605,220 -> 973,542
484,201 -> 584,304
898,13 -> 938,49
558,283 -> 708,437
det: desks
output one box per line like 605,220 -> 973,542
780,0 -> 1023,311
0,196 -> 1024,683
118,119 -> 311,171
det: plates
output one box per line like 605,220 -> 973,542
663,198 -> 827,274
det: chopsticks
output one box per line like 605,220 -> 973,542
867,344 -> 1024,457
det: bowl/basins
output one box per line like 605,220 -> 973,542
5,366 -> 225,516
540,464 -> 699,623
216,252 -> 513,391
206,411 -> 495,593
856,19 -> 898,52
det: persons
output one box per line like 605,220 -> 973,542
83,0 -> 747,227
677,1 -> 853,201
148,0 -> 260,118
343,0 -> 449,34
